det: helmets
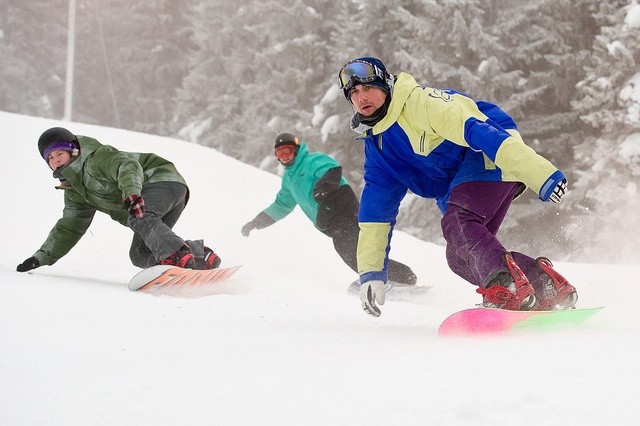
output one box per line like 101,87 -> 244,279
38,127 -> 78,157
275,132 -> 298,148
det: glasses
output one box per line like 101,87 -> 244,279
275,145 -> 296,158
338,59 -> 390,90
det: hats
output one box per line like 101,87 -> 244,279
339,57 -> 391,101
43,138 -> 79,165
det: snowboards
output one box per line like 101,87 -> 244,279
437,306 -> 604,340
348,278 -> 433,303
128,264 -> 243,294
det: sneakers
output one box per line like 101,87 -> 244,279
474,280 -> 539,310
537,277 -> 579,311
160,242 -> 198,271
195,245 -> 221,270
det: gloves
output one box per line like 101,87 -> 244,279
543,176 -> 569,202
16,257 -> 40,272
359,277 -> 385,317
124,194 -> 146,219
241,220 -> 255,237
313,188 -> 328,205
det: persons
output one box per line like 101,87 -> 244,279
240,132 -> 417,285
16,126 -> 221,273
338,57 -> 578,317
